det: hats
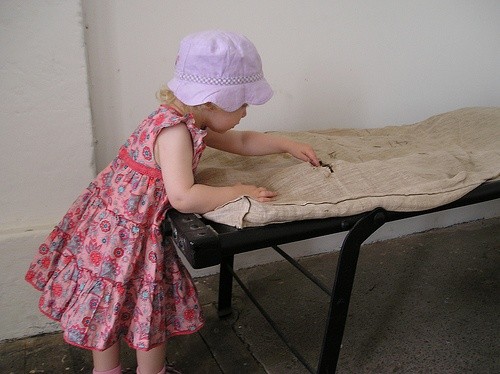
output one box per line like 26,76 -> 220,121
168,31 -> 273,112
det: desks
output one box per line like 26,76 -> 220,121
165,104 -> 500,374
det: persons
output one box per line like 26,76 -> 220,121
24,29 -> 321,374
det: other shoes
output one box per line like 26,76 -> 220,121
136,364 -> 166,374
93,363 -> 122,374
